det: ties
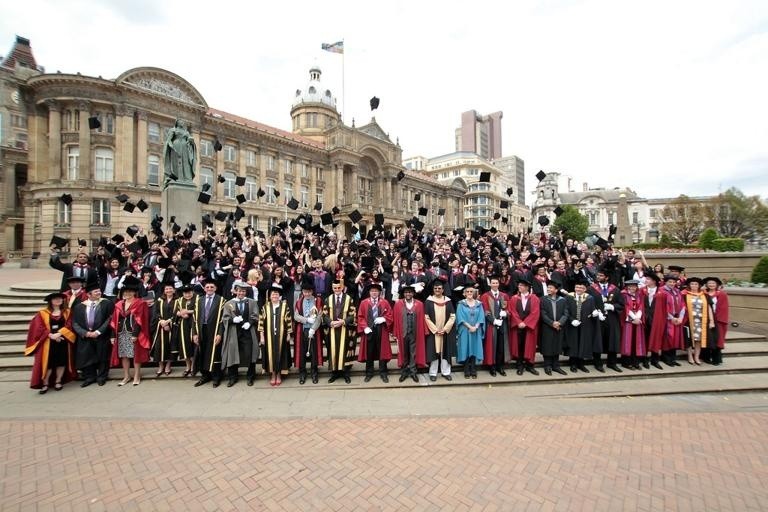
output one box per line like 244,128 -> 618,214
601,284 -> 608,301
372,299 -> 379,322
576,295 -> 583,320
670,289 -> 679,315
89,301 -> 96,331
335,296 -> 343,318
304,298 -> 311,324
238,298 -> 244,321
203,295 -> 212,325
493,293 -> 500,319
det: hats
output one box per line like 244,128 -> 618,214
49,193 -> 616,294
274,189 -> 280,197
217,175 -> 226,183
479,172 -> 491,182
395,170 -> 406,183
235,176 -> 247,186
623,264 -> 723,289
369,96 -> 381,111
257,187 -> 266,198
536,170 -> 546,182
507,187 -> 513,196
214,140 -> 222,152
202,183 -> 210,192
88,116 -> 101,130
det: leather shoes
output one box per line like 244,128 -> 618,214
39,370 -> 107,395
261,366 -> 351,386
156,366 -> 252,387
364,372 -> 452,382
571,360 -> 681,373
464,365 -> 567,379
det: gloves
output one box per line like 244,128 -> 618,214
571,319 -> 581,327
363,317 -> 386,335
232,315 -> 251,331
592,302 -> 615,321
306,315 -> 316,339
493,309 -> 507,327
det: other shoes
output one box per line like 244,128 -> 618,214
118,374 -> 141,386
687,357 -> 721,366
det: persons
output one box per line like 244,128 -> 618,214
160,114 -> 198,189
22,221 -> 729,395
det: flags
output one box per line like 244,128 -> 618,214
321,40 -> 345,55
